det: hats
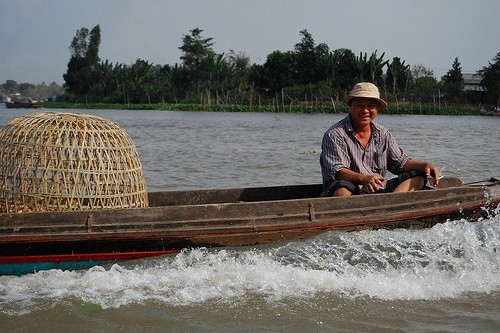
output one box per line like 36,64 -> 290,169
344,82 -> 387,109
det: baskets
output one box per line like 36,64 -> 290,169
1,113 -> 150,214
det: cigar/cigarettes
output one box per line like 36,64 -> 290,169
435,176 -> 443,180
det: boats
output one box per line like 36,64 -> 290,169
5,100 -> 43,109
0,175 -> 500,277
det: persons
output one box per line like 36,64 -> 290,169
319,83 -> 441,197
